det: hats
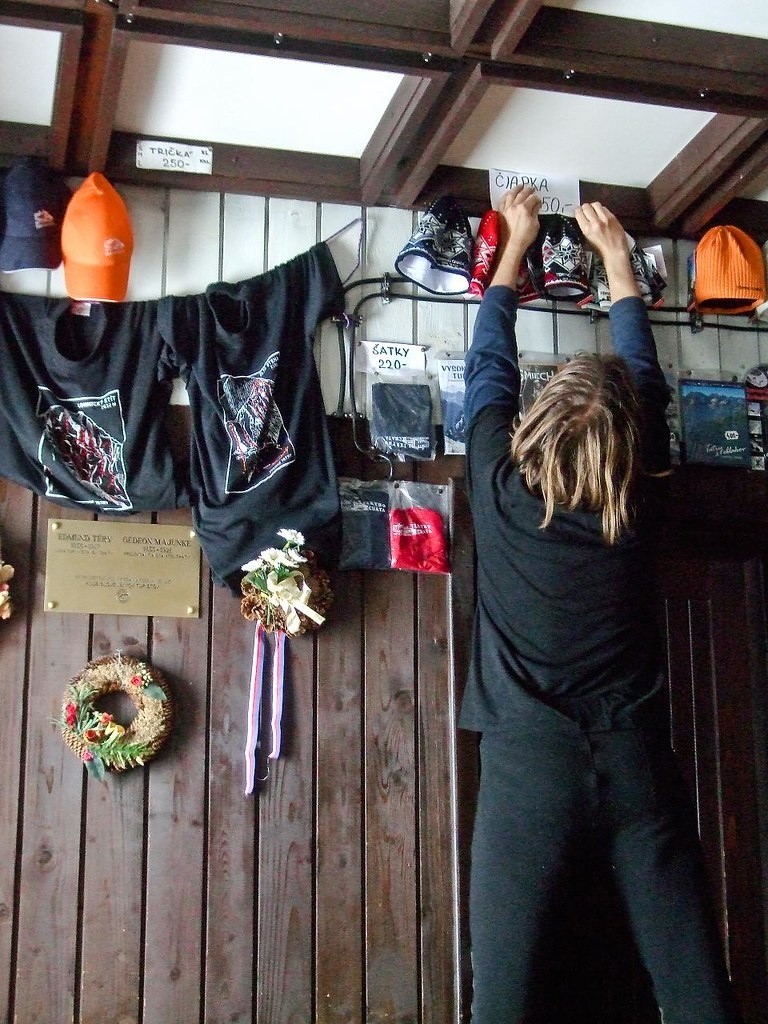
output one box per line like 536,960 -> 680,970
694,224 -> 767,314
0,156 -> 73,273
755,240 -> 768,322
468,209 -> 540,303
394,194 -> 474,296
60,171 -> 134,302
526,214 -> 591,302
590,230 -> 668,310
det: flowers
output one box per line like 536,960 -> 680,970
49,647 -> 168,781
241,532 -> 316,624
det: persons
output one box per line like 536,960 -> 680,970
456,183 -> 733,1024
